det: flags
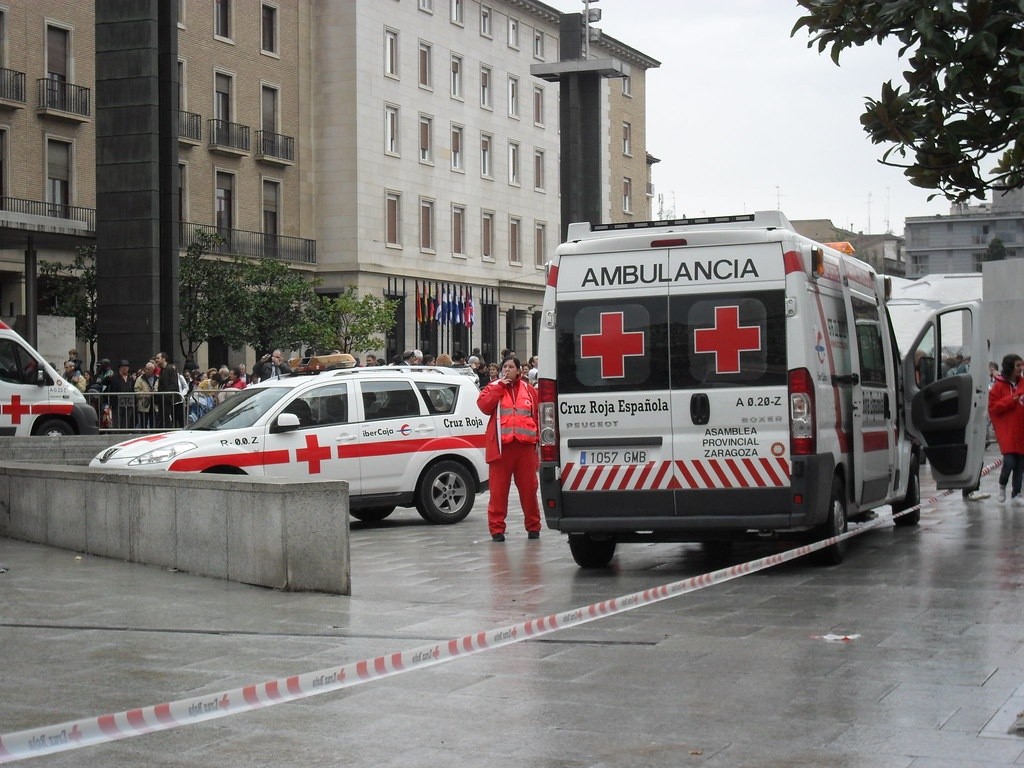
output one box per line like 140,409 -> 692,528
416,282 -> 475,328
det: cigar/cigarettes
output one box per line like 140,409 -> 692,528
502,373 -> 505,375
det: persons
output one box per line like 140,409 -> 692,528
988,354 -> 1024,506
962,461 -> 991,500
914,350 -> 1002,449
476,356 -> 541,542
11,347 -> 537,435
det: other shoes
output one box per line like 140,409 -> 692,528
1011,493 -> 1024,507
528,531 -> 539,539
492,534 -> 504,541
997,483 -> 1006,502
968,491 -> 991,500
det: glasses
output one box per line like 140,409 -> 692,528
366,360 -> 371,362
64,367 -> 69,369
490,369 -> 497,371
273,356 -> 282,359
146,368 -> 151,371
156,356 -> 161,359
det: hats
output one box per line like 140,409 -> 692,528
118,360 -> 130,366
403,350 -> 413,357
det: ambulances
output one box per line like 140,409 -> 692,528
0,318 -> 100,437
85,353 -> 492,526
535,210 -> 989,567
